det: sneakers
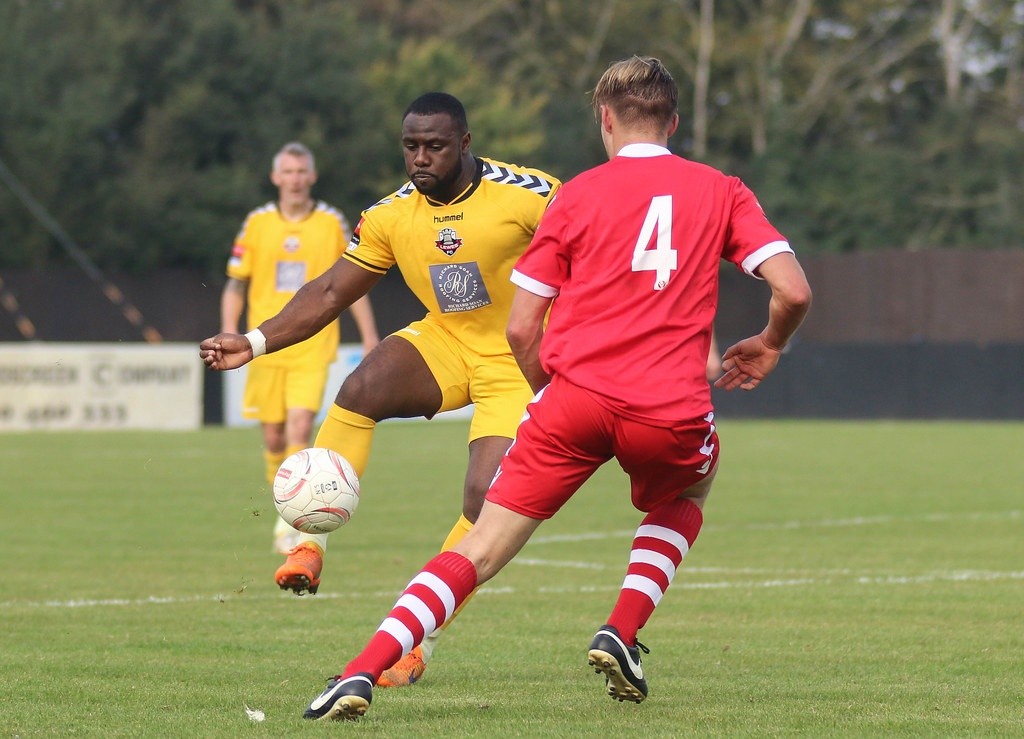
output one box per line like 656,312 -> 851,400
377,645 -> 425,687
589,625 -> 651,705
304,672 -> 374,722
275,541 -> 323,596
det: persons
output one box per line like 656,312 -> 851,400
219,142 -> 381,552
200,92 -> 564,686
301,56 -> 813,719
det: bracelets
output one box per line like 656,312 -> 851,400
245,328 -> 267,360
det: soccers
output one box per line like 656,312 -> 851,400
273,447 -> 361,535
